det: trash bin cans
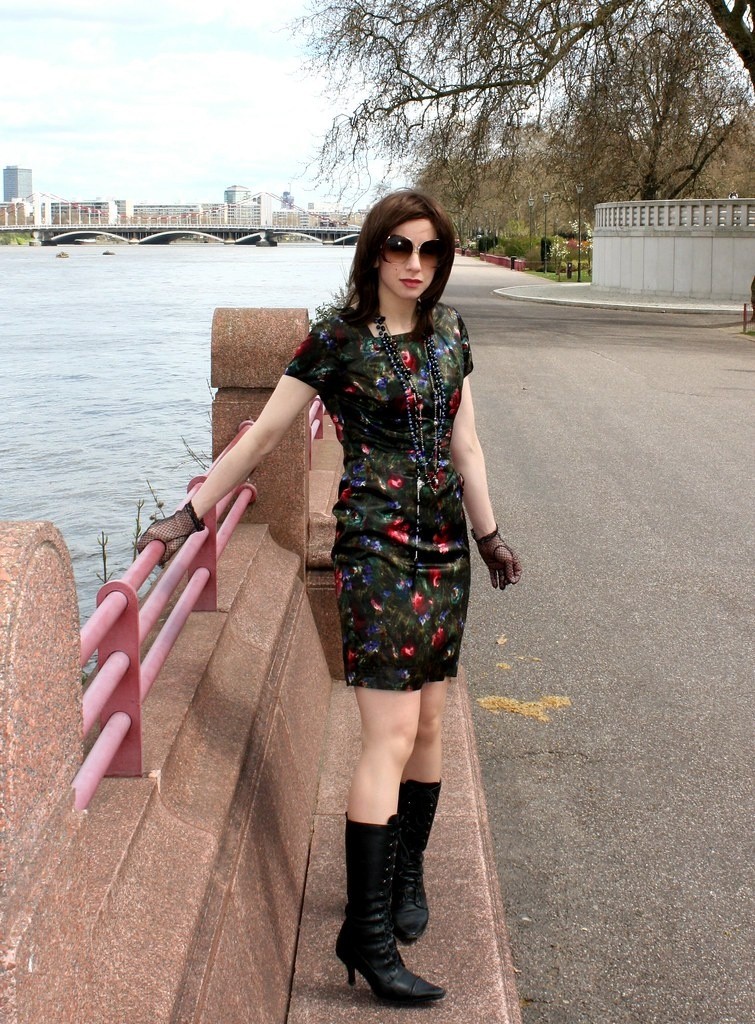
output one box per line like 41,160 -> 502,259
511,256 -> 516,270
461,248 -> 465,256
567,262 -> 572,277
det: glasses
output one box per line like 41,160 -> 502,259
379,234 -> 446,267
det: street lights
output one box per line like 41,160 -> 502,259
528,198 -> 535,271
575,181 -> 585,282
543,192 -> 550,274
485,213 -> 488,254
492,210 -> 497,256
475,215 -> 479,256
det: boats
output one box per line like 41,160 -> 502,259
102,250 -> 116,256
56,251 -> 70,259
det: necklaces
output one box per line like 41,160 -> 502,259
373,313 -> 446,495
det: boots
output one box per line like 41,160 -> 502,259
392,778 -> 444,940
336,811 -> 445,1006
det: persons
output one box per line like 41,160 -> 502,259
135,192 -> 522,1007
